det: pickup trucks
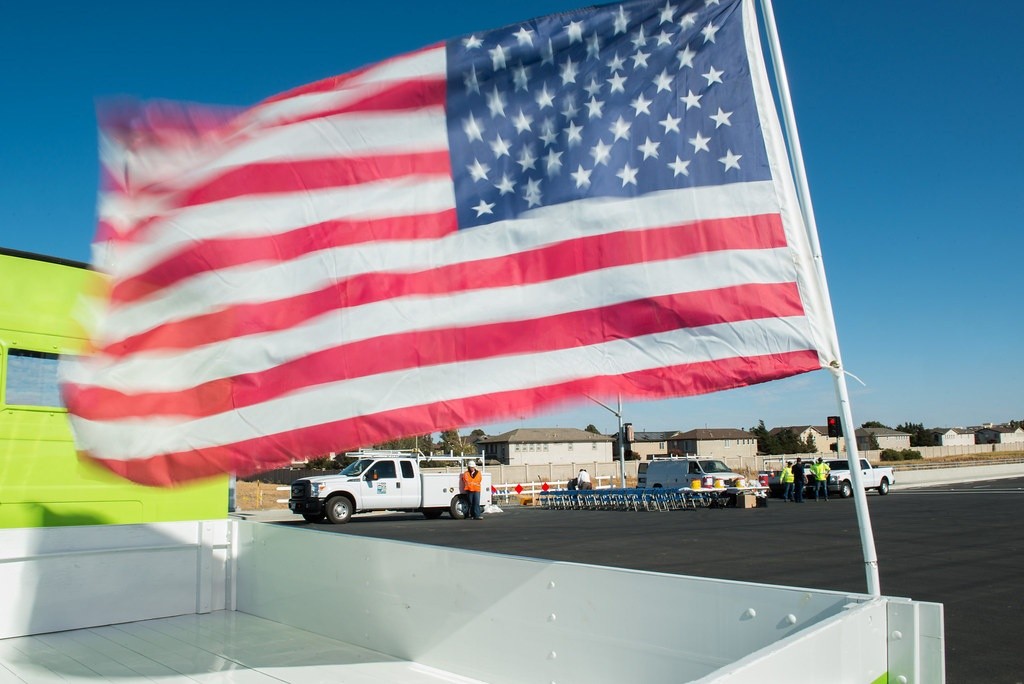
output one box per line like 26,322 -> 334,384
759,457 -> 896,498
288,448 -> 492,524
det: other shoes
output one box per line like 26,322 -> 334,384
784,498 -> 794,502
815,497 -> 819,502
469,515 -> 483,520
825,497 -> 828,502
796,499 -> 804,503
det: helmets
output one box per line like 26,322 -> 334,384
467,461 -> 476,467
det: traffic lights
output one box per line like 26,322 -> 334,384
828,418 -> 838,437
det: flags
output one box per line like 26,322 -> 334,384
60,0 -> 840,490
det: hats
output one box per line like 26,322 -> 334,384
788,461 -> 792,464
818,458 -> 823,461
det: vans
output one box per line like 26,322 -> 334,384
637,453 -> 744,488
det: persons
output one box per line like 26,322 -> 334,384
568,469 -> 592,505
463,460 -> 483,520
779,457 -> 830,502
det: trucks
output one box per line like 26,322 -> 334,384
0,248 -> 947,684
